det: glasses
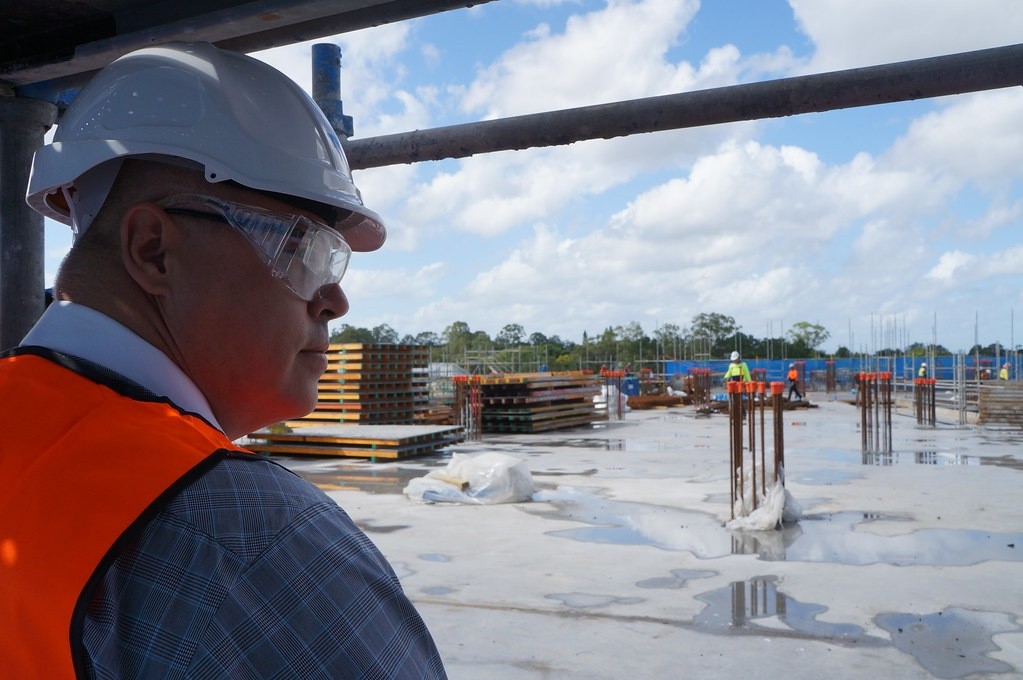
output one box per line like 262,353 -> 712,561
136,194 -> 354,303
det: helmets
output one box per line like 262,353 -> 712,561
789,363 -> 795,368
731,351 -> 739,360
921,362 -> 927,367
24,40 -> 387,252
1003,363 -> 1011,369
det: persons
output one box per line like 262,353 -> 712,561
724,351 -> 752,419
787,363 -> 801,401
0,40 -> 449,679
918,363 -> 927,378
1000,365 -> 1008,380
